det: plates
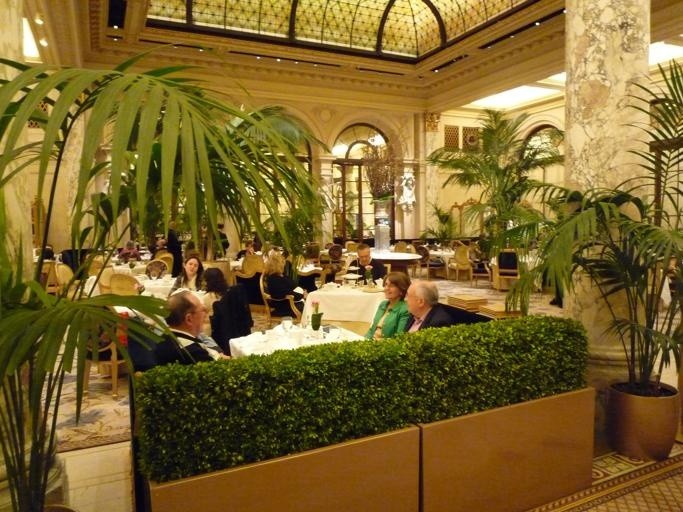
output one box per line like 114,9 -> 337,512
341,272 -> 362,280
342,266 -> 360,271
195,290 -> 206,296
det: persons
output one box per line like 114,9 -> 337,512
204,267 -> 229,336
365,271 -> 451,340
119,240 -> 142,263
264,252 -> 309,318
205,220 -> 230,260
237,241 -> 263,261
148,220 -> 184,279
148,290 -> 231,366
167,257 -> 208,296
346,242 -> 387,285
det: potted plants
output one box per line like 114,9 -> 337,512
427,56 -> 683,463
130,316 -> 597,511
1,43 -> 341,512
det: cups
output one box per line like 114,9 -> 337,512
323,279 -> 384,293
161,274 -> 172,285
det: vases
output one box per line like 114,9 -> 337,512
372,198 -> 392,250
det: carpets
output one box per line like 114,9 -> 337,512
44,262 -> 682,454
526,452 -> 683,511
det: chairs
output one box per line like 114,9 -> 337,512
35,241 -> 235,400
235,241 -> 521,330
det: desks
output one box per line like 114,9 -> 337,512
347,250 -> 424,278
301,282 -> 402,326
428,249 -> 458,280
229,318 -> 373,365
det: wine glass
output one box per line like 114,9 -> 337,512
247,314 -> 341,353
314,277 -> 322,293
119,258 -> 125,268
305,259 -> 314,270
150,270 -> 158,286
